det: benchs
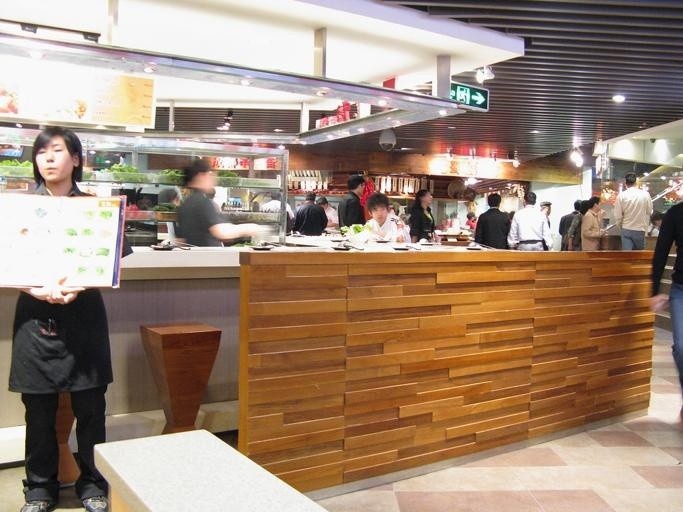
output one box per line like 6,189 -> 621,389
93,429 -> 328,511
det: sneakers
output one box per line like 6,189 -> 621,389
80,492 -> 108,511
18,498 -> 56,512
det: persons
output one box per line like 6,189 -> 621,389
581,197 -> 607,251
540,202 -> 551,251
158,189 -> 180,208
651,211 -> 663,237
259,191 -> 294,236
296,190 -> 328,236
410,190 -> 435,243
507,191 -> 553,252
338,175 -> 366,234
474,194 -> 511,250
174,162 -> 266,247
364,193 -> 410,243
567,200 -> 588,251
615,173 -> 653,250
316,195 -> 338,228
8,128 -> 115,512
650,202 -> 682,428
466,212 -> 477,229
559,200 -> 583,251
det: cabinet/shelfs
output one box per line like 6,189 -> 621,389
0,126 -> 290,246
293,193 -> 482,239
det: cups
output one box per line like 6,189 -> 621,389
330,228 -> 335,238
336,228 -> 341,238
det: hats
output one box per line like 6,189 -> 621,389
184,159 -> 221,175
539,200 -> 551,208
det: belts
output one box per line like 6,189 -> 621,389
518,238 -> 545,245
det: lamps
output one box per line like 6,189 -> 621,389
514,149 -> 520,168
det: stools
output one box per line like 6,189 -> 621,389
141,325 -> 222,436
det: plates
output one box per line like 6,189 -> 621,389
466,248 -> 481,251
420,243 -> 433,246
376,240 -> 391,243
250,247 -> 273,251
331,247 -> 352,251
330,240 -> 343,243
151,246 -> 175,251
393,247 -> 410,251
